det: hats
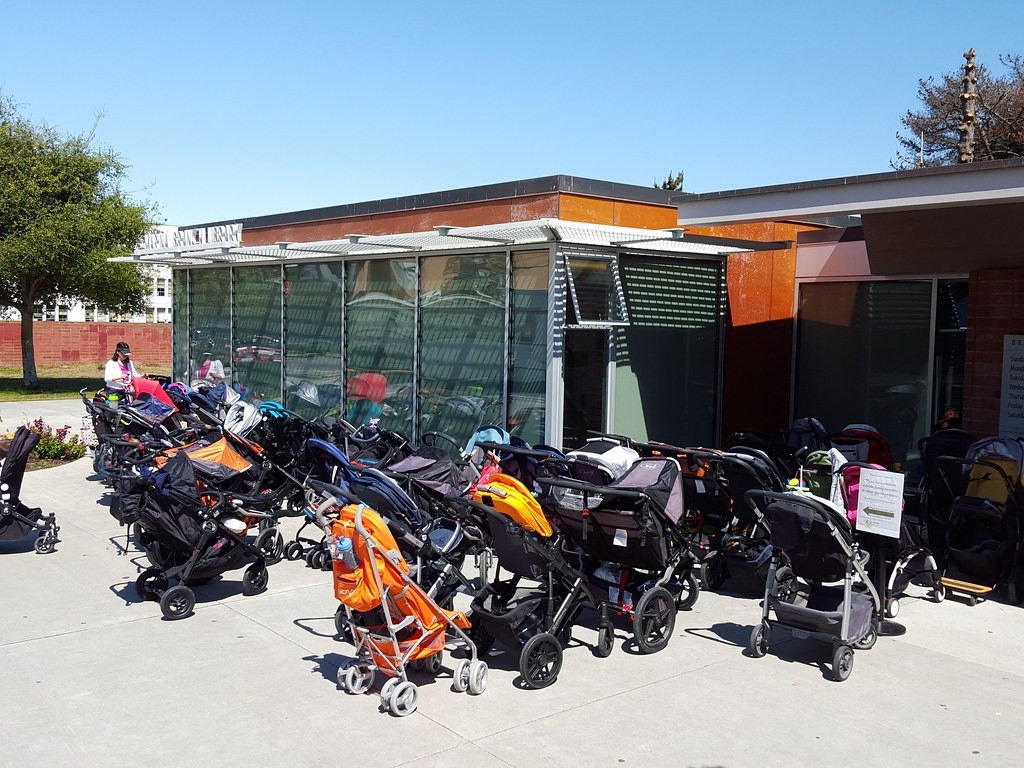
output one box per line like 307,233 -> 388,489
117,342 -> 132,356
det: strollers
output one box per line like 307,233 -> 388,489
77,373 -> 280,619
1,427 -> 60,553
281,370 -> 510,448
282,411 -> 1024,717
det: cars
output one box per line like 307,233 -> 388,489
191,324 -> 234,367
235,335 -> 284,368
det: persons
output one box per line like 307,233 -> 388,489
103,341 -> 149,399
183,344 -> 230,387
930,406 -> 961,435
